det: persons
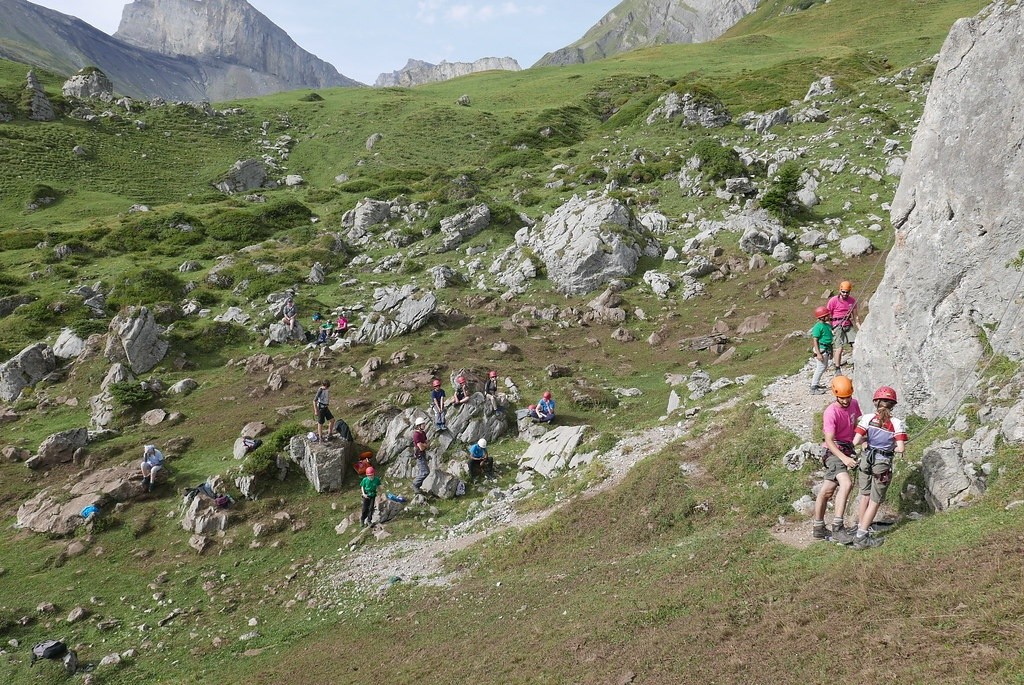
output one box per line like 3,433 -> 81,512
431,379 -> 447,432
813,378 -> 862,545
414,417 -> 431,494
319,314 -> 349,338
282,298 -> 298,336
141,446 -> 164,493
810,306 -> 833,395
484,371 -> 500,416
360,467 -> 384,527
453,376 -> 471,407
469,439 -> 494,483
313,379 -> 338,444
852,386 -> 908,550
827,281 -> 862,377
532,391 -> 556,424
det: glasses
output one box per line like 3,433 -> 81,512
842,290 -> 850,294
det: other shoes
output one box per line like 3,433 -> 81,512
366,520 -> 373,528
834,370 -> 842,376
548,419 -> 550,424
319,434 -> 338,446
531,418 -> 541,423
496,410 -> 500,416
810,387 -> 826,395
410,484 -> 421,494
453,403 -> 457,407
435,424 -> 447,432
142,477 -> 156,492
360,518 -> 364,527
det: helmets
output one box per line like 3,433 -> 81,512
543,392 -> 551,399
489,371 -> 497,378
457,377 -> 466,383
840,281 -> 851,291
415,418 -> 424,425
814,306 -> 831,319
529,405 -> 536,410
832,376 -> 855,397
478,438 -> 487,449
366,467 -> 375,475
433,380 -> 441,386
872,386 -> 897,404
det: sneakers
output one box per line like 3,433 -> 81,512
832,521 -> 853,544
813,524 -> 830,540
855,533 -> 884,549
